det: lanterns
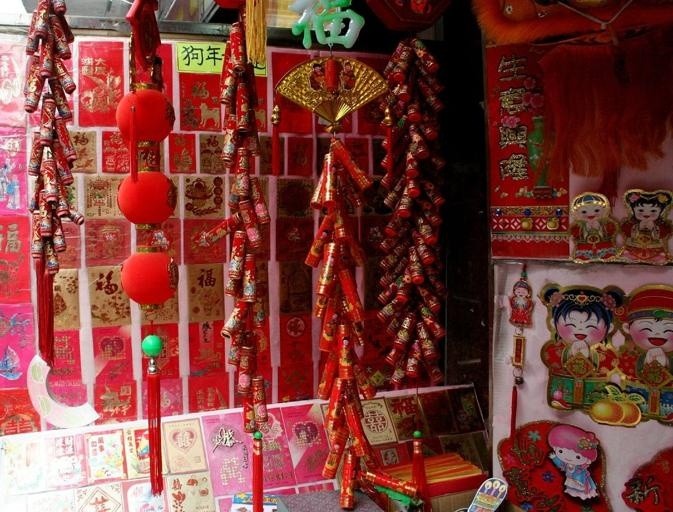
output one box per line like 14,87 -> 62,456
115,77 -> 178,495
